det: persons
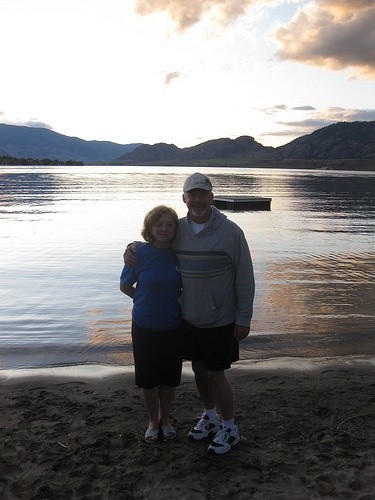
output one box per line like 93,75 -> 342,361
125,170 -> 256,456
120,203 -> 188,444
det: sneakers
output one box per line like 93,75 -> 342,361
187,412 -> 222,441
206,427 -> 243,456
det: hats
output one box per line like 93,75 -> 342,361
181,172 -> 213,193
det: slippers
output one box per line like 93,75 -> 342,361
159,420 -> 177,440
144,424 -> 160,443
